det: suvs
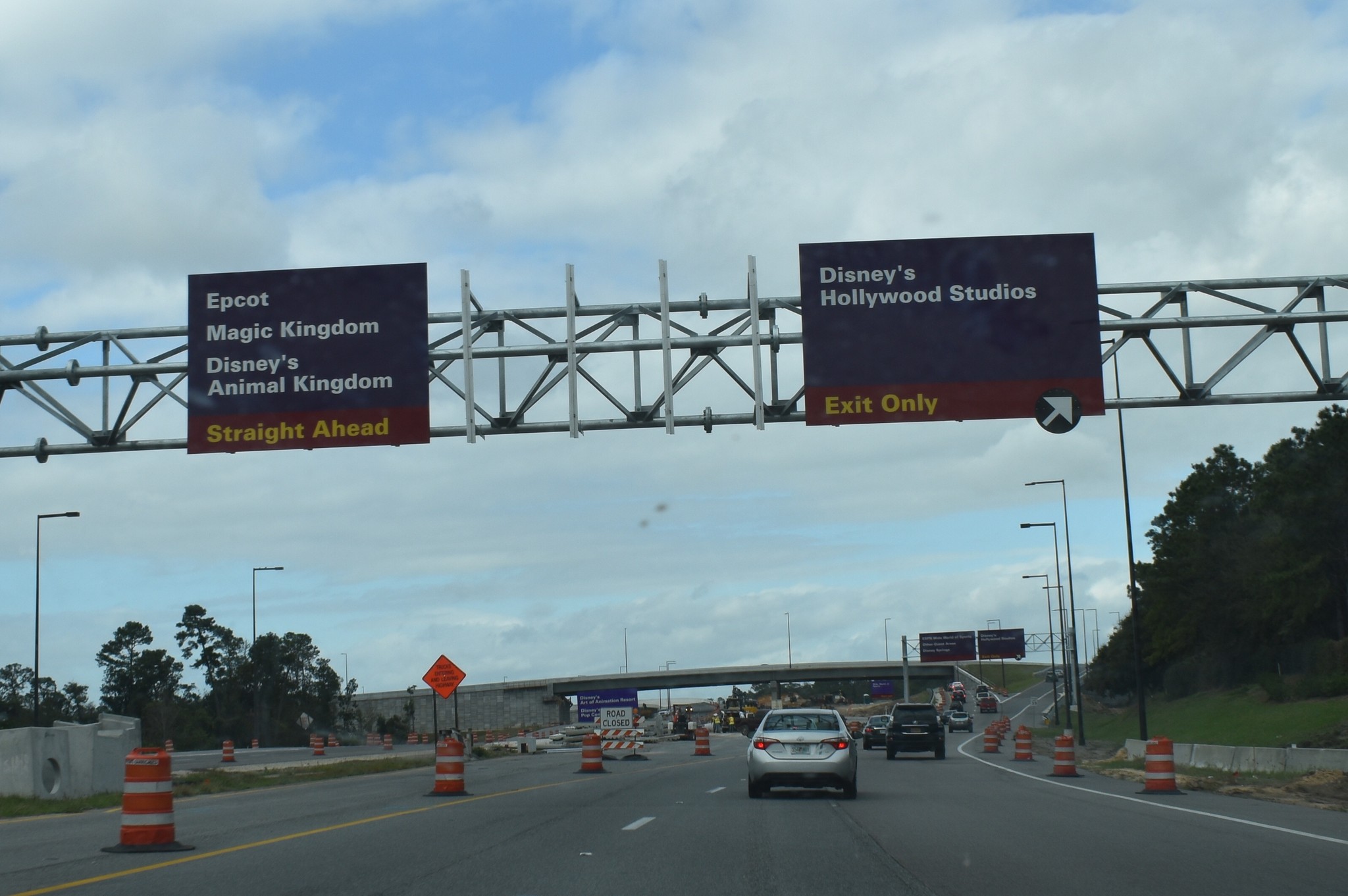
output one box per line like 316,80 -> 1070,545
975,685 -> 998,713
881,703 -> 947,759
948,711 -> 973,733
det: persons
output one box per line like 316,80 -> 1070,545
710,712 -> 734,734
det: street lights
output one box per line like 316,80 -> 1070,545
659,661 -> 677,710
34,512 -> 80,726
253,567 -> 284,643
1019,480 -> 1084,745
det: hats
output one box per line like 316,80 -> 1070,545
713,713 -> 717,716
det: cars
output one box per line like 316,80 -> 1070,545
942,682 -> 966,724
862,715 -> 891,750
658,709 -> 670,717
747,708 -> 864,799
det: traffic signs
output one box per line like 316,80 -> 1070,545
799,234 -> 1106,434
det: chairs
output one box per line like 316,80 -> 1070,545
807,721 -> 830,730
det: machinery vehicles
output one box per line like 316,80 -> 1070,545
719,698 -> 758,724
